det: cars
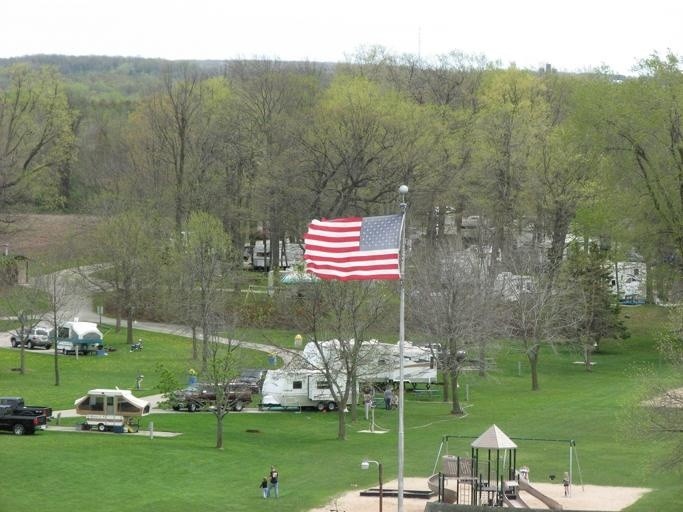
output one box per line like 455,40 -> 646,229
229,369 -> 267,394
10,328 -> 53,350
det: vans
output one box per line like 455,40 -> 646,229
171,382 -> 252,412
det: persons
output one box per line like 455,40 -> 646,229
560,472 -> 570,497
266,467 -> 279,500
383,386 -> 399,411
259,477 -> 272,500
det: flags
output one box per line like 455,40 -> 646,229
303,213 -> 401,281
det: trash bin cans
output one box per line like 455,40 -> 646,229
268,356 -> 277,366
295,335 -> 303,346
188,369 -> 198,384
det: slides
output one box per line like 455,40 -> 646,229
426,472 -> 563,511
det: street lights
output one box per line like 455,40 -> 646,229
360,460 -> 383,512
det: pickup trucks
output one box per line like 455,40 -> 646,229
0,398 -> 53,436
424,343 -> 466,363
